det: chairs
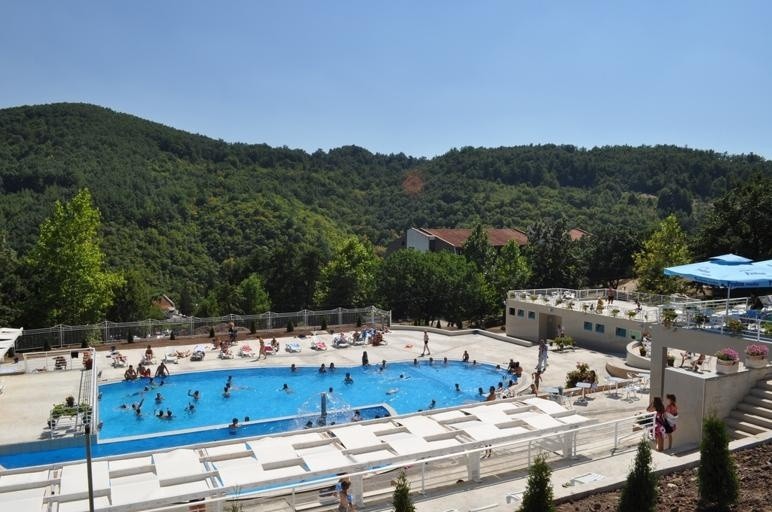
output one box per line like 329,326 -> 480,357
604,371 -> 650,403
692,355 -> 713,372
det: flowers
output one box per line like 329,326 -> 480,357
745,344 -> 768,355
714,347 -> 738,360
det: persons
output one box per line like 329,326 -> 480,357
679,351 -> 695,368
646,397 -> 666,452
483,443 -> 492,458
597,298 -> 603,313
662,394 -> 678,450
336,472 -> 350,493
189,497 -> 206,512
746,292 -> 763,324
607,285 -> 616,305
690,354 -> 705,371
557,324 -> 565,338
639,333 -> 647,356
110,320 -> 548,435
338,480 -> 356,512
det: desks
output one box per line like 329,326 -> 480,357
544,382 -> 592,405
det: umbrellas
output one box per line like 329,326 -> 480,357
752,260 -> 772,270
662,253 -> 772,328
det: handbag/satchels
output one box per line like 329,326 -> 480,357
662,419 -> 672,433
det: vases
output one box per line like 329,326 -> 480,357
746,355 -> 767,368
717,359 -> 739,374
